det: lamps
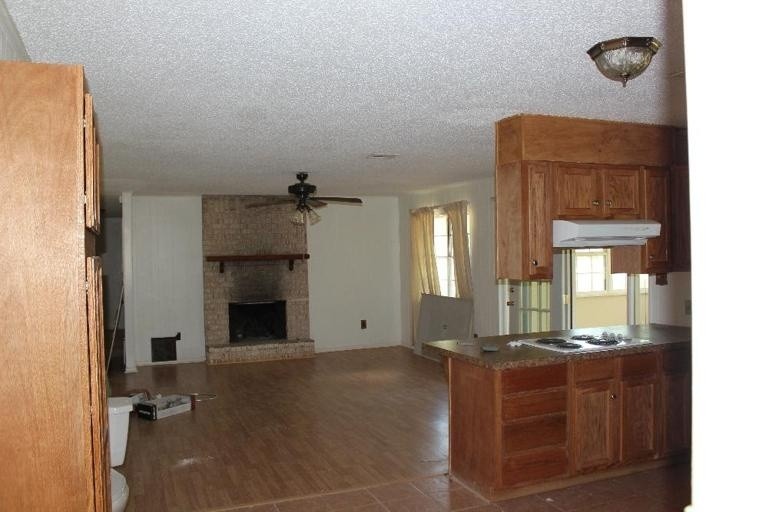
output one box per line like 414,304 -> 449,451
586,35 -> 662,87
283,202 -> 327,224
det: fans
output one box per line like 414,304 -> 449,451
246,172 -> 362,210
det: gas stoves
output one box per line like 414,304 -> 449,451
520,333 -> 653,353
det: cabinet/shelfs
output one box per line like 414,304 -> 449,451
496,115 -> 689,281
446,347 -> 692,504
0,60 -> 112,511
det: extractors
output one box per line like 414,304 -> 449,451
552,219 -> 660,248
